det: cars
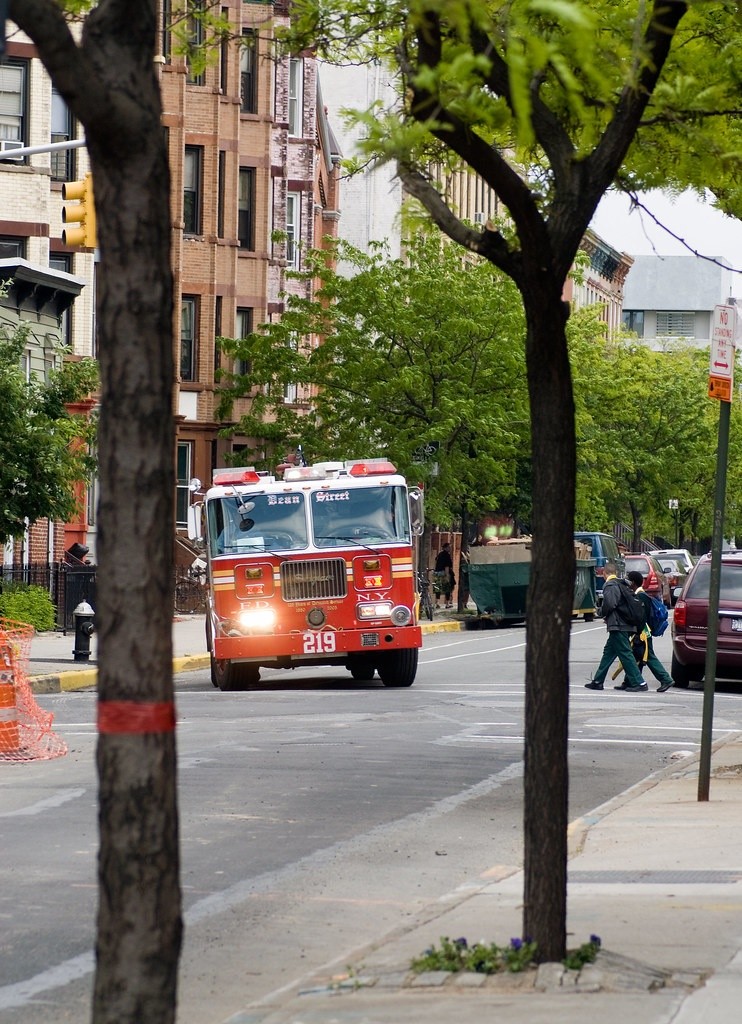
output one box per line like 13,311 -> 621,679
623,547 -> 742,688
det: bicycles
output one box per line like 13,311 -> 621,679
414,567 -> 436,621
174,572 -> 206,614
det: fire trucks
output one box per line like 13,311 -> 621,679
186,456 -> 425,691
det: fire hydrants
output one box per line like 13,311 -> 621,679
71,599 -> 95,662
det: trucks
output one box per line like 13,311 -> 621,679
449,530 -> 628,630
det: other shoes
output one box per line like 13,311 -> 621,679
446,605 -> 453,608
436,605 -> 440,608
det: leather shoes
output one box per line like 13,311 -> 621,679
657,681 -> 675,692
585,680 -> 604,690
614,682 -> 625,690
626,683 -> 649,692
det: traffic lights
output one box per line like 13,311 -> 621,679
59,170 -> 98,250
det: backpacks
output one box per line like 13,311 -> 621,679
639,591 -> 668,637
614,581 -> 641,626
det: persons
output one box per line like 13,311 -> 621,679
461,541 -> 473,565
472,534 -> 485,546
434,542 -> 455,609
585,563 -> 675,694
